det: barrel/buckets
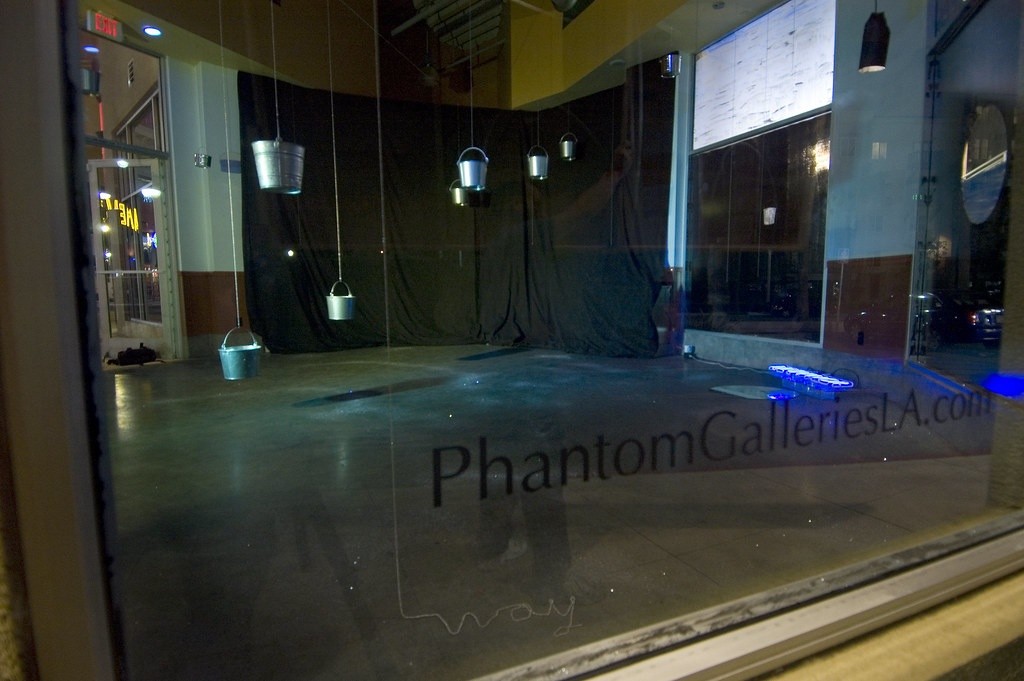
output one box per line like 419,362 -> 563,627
527,144 -> 549,180
250,137 -> 306,195
449,178 -> 469,208
325,279 -> 356,321
456,147 -> 490,191
217,325 -> 262,381
659,53 -> 682,78
559,132 -> 578,162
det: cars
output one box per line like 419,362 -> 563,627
917,291 -> 1006,347
